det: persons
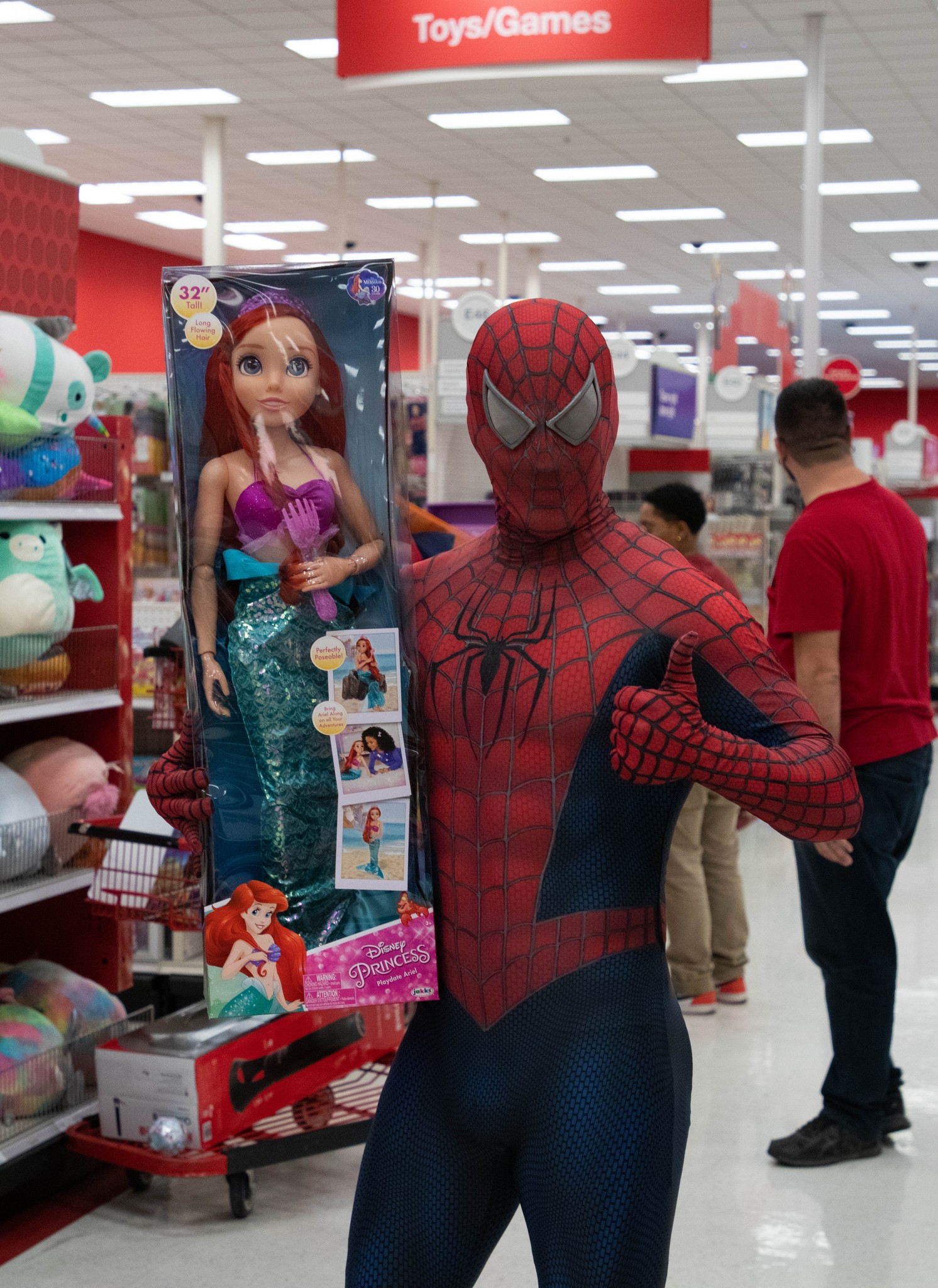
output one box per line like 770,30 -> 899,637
765,375 -> 935,1166
147,297 -> 865,1288
641,482 -> 758,1014
193,285 -> 391,950
359,726 -> 405,775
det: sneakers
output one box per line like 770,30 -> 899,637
768,1114 -> 881,1166
715,978 -> 747,1003
678,992 -> 717,1015
882,1084 -> 912,1135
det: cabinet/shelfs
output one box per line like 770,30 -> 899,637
0,413 -> 133,1266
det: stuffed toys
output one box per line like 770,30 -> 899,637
1,306 -> 164,1120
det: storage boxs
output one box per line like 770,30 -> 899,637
96,1000 -> 404,1150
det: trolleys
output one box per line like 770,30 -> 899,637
143,645 -> 186,731
58,816 -> 419,1222
